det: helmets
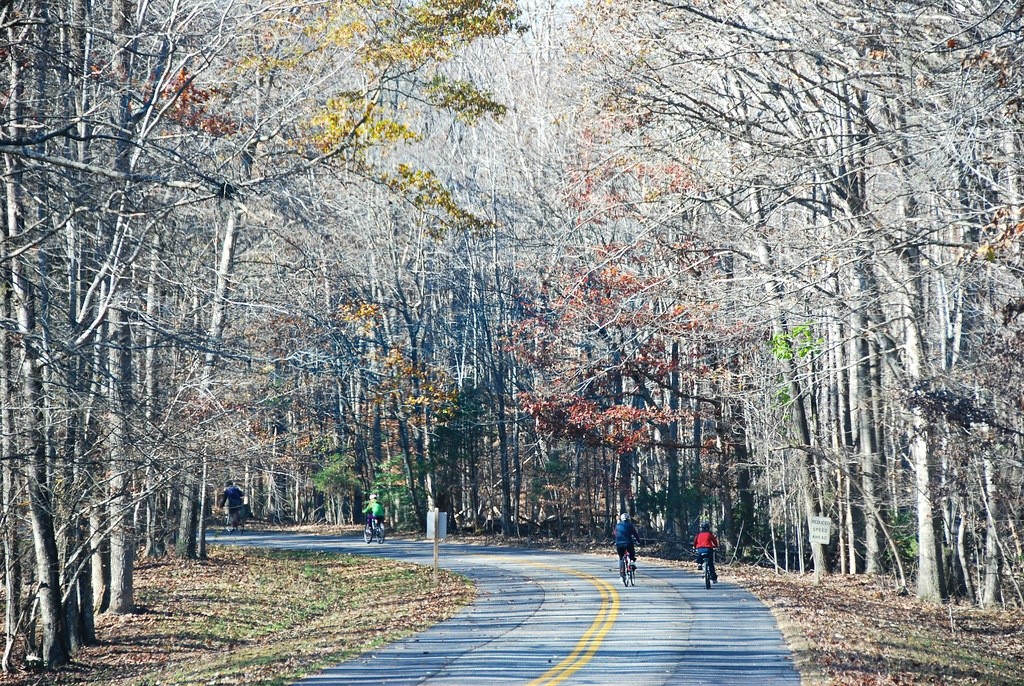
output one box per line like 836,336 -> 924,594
621,513 -> 630,520
699,522 -> 710,528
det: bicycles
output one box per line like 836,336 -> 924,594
693,546 -> 718,589
363,512 -> 385,544
220,504 -> 246,536
619,542 -> 639,586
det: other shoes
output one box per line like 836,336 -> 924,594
698,564 -> 702,570
714,575 -> 717,582
621,577 -> 627,582
630,562 -> 637,569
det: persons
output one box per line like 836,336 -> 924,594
693,523 -> 720,584
615,513 -> 642,578
220,482 -> 244,535
362,494 -> 385,535
